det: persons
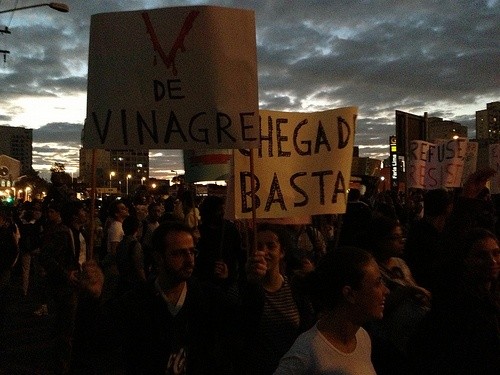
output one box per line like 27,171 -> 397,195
104,218 -> 228,375
269,245 -> 389,375
433,226 -> 500,375
1,167 -> 499,321
355,216 -> 427,348
222,221 -> 315,375
